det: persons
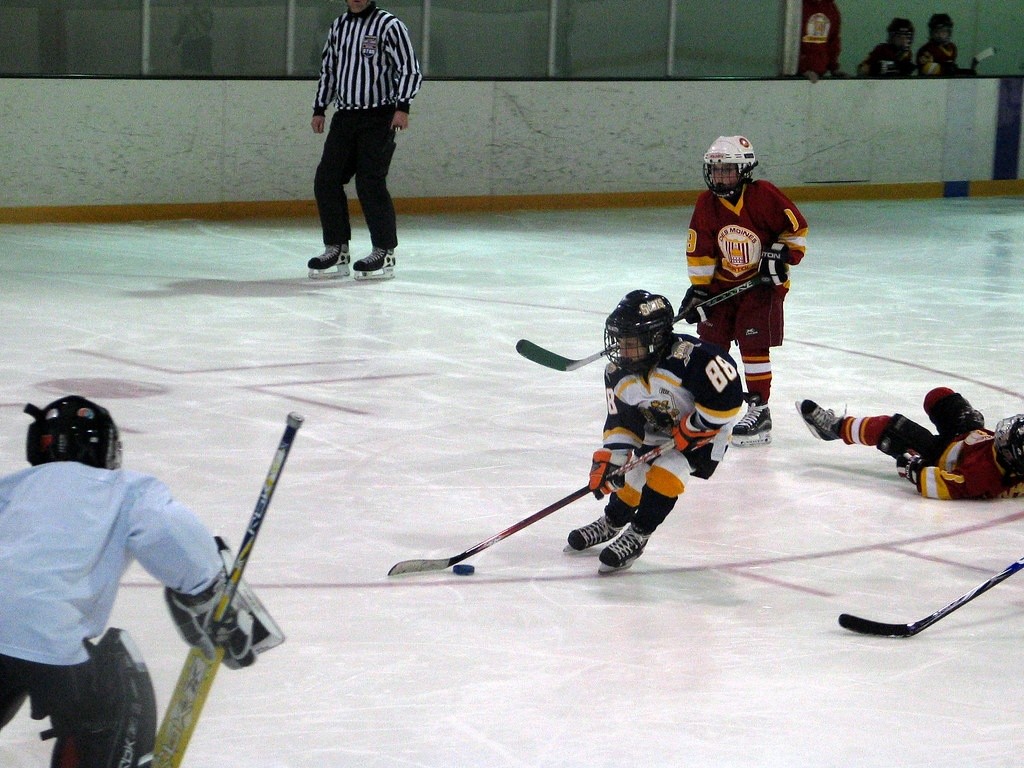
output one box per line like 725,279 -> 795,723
915,13 -> 976,76
562,291 -> 744,575
149,0 -> 215,77
675,134 -> 808,451
795,387 -> 1024,500
796,0 -> 850,83
305,0 -> 422,282
0,397 -> 256,767
857,18 -> 917,78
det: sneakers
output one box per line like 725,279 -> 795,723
732,392 -> 772,446
795,399 -> 847,441
308,241 -> 351,279
353,246 -> 396,280
563,515 -> 623,552
598,522 -> 651,575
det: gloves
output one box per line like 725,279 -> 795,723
176,574 -> 254,660
589,448 -> 633,500
671,409 -> 720,453
758,242 -> 789,291
678,285 -> 712,324
896,448 -> 929,493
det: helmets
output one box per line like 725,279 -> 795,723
703,136 -> 758,198
604,289 -> 674,371
994,414 -> 1024,485
24,395 -> 123,470
929,14 -> 954,44
886,18 -> 914,54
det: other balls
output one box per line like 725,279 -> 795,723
453,565 -> 474,575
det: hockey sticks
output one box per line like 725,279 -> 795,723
388,440 -> 677,577
970,46 -> 996,70
516,278 -> 761,372
838,555 -> 1023,637
154,413 -> 307,768
